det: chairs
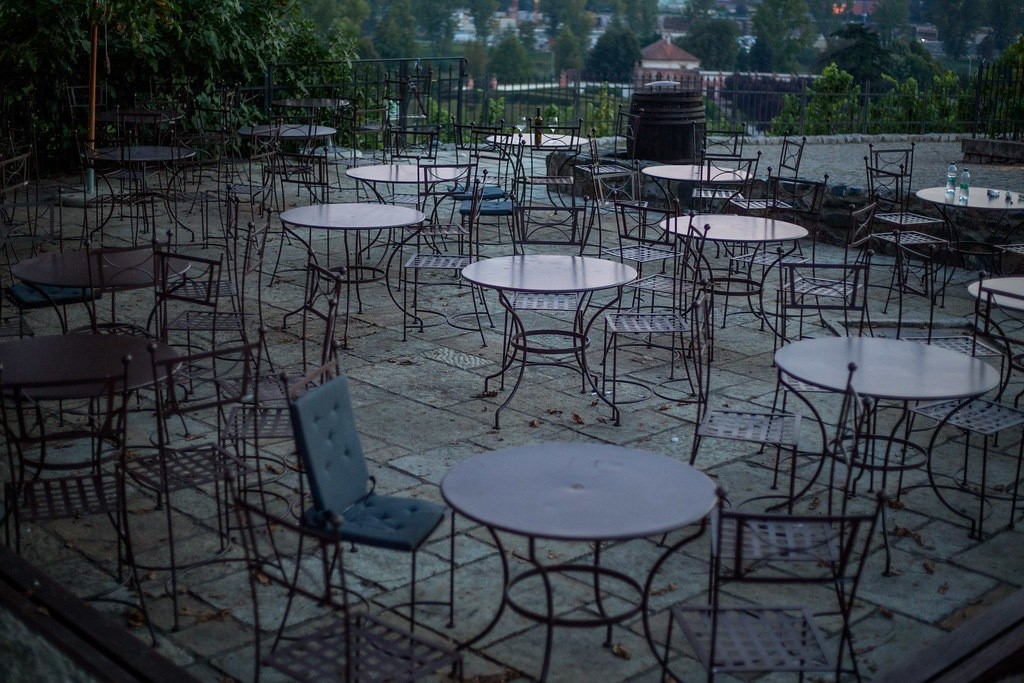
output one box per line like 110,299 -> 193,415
0,61 -> 1023,682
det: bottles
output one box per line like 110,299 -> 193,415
959,167 -> 971,200
535,106 -> 542,144
945,161 -> 958,193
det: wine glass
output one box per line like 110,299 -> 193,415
548,115 -> 559,137
515,115 -> 527,133
